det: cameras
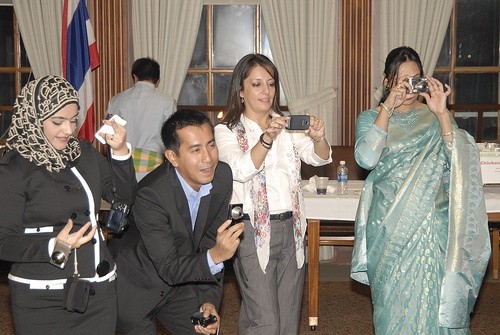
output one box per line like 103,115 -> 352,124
405,76 -> 429,93
190,311 -> 217,327
227,203 -> 245,239
48,238 -> 72,269
104,200 -> 131,235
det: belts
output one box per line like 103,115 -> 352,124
240,211 -> 293,222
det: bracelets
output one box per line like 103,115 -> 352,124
441,132 -> 453,137
258,133 -> 274,150
312,135 -> 326,142
379,102 -> 393,119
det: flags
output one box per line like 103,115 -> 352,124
60,0 -> 101,144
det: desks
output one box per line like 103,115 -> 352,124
301,180 -> 500,330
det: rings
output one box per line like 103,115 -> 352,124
397,83 -> 403,89
110,135 -> 115,140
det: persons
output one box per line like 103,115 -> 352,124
214,52 -> 332,335
0,74 -> 138,335
348,47 -> 492,335
104,57 -> 178,183
113,109 -> 245,335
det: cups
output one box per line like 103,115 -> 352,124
315,177 -> 329,195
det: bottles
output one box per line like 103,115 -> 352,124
336,161 -> 348,195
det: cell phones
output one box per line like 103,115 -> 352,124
95,114 -> 127,144
285,115 -> 310,130
